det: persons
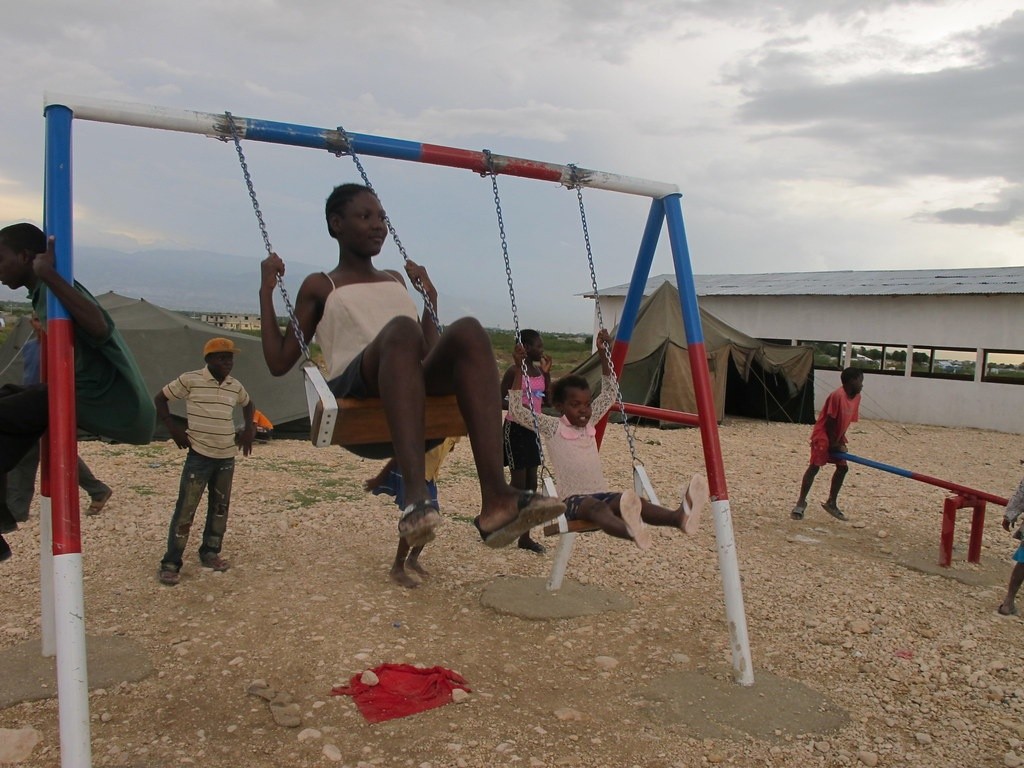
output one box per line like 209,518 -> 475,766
260,184 -> 566,546
790,364 -> 865,521
501,327 -> 554,554
154,338 -> 254,585
998,478 -> 1024,615
507,328 -> 706,553
0,224 -> 143,559
361,436 -> 466,591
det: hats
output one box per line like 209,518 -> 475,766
203,337 -> 241,361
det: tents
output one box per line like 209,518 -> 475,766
3,288 -> 276,423
567,279 -> 817,429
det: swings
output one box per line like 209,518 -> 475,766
231,137 -> 469,447
490,172 -> 660,537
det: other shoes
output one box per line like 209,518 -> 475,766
517,539 -> 546,552
199,552 -> 230,570
159,560 -> 181,584
86,486 -> 112,515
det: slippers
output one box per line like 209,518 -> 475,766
485,490 -> 567,548
790,501 -> 807,520
998,602 -> 1016,614
821,503 -> 848,521
398,499 -> 439,547
683,473 -> 708,536
619,489 -> 652,550
